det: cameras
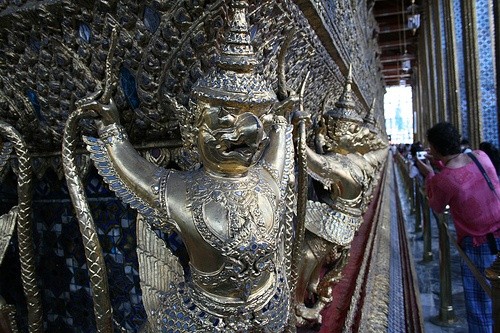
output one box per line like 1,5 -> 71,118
417,151 -> 427,160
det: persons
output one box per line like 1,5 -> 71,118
84,108 -> 392,332
395,123 -> 500,333
